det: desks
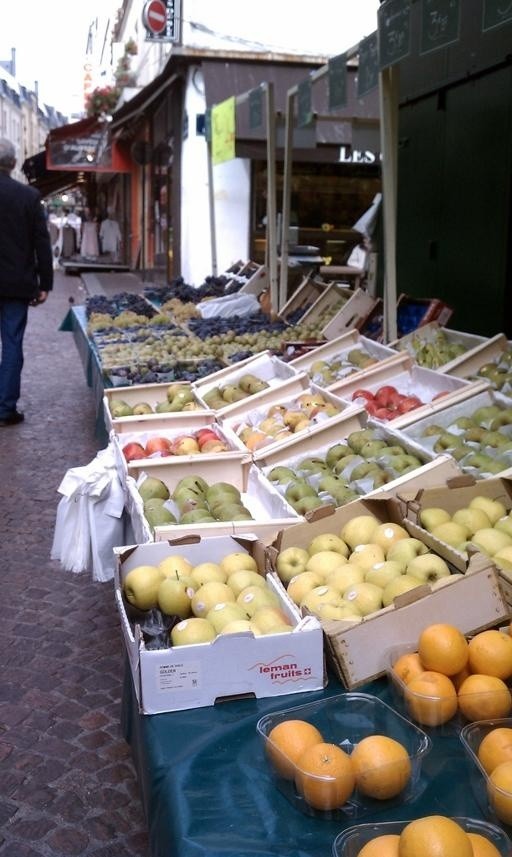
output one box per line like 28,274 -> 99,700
57,307 -> 111,429
125,663 -> 512,854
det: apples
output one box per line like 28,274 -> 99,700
271,514 -> 453,623
234,388 -> 340,453
411,497 -> 512,572
121,552 -> 289,643
229,394 -> 332,453
125,426 -> 230,466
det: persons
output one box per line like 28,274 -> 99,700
38,201 -> 124,265
0,137 -> 55,425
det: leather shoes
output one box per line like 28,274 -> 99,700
1,410 -> 24,428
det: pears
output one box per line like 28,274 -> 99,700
201,375 -> 270,411
474,339 -> 512,398
419,400 -> 510,478
264,429 -> 424,517
137,478 -> 252,529
404,330 -> 468,369
308,350 -> 379,386
111,382 -> 199,416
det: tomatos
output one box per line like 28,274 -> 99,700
352,381 -> 449,423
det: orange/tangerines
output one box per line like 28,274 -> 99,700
478,728 -> 512,826
266,720 -> 324,781
294,743 -> 355,810
393,622 -> 512,726
358,816 -> 502,856
350,735 -> 412,800
